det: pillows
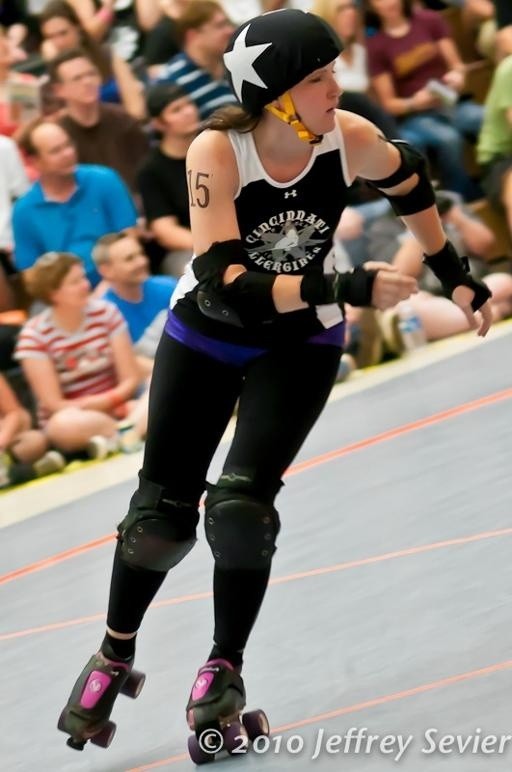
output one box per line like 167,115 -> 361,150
222,8 -> 343,112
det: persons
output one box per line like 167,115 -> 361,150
1,1 -> 511,487
56,6 -> 491,765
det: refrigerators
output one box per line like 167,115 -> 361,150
57,637 -> 146,752
186,648 -> 270,766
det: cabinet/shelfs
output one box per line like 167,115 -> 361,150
396,296 -> 427,354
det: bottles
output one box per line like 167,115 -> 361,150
145,83 -> 188,116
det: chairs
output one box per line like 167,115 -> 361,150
32,449 -> 66,476
87,434 -> 109,460
